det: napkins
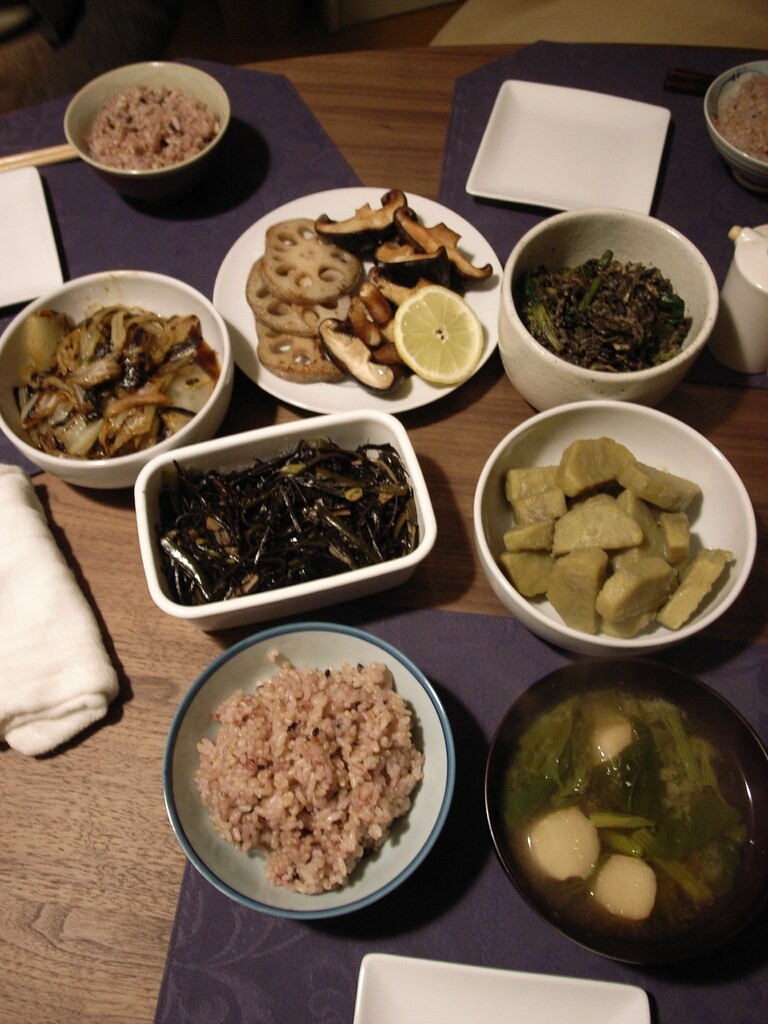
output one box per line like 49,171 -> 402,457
0,463 -> 119,756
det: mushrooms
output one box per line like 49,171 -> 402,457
313,186 -> 493,394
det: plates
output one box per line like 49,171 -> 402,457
465,79 -> 671,216
354,953 -> 650,1024
212,187 -> 504,414
160,622 -> 456,920
0,166 -> 64,306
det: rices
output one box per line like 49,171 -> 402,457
85,85 -> 220,172
711,70 -> 768,164
193,648 -> 425,894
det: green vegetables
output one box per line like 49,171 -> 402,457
519,248 -> 689,370
502,701 -> 745,909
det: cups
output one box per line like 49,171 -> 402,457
707,224 -> 768,373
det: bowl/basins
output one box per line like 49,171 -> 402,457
134,409 -> 438,632
472,400 -> 757,658
703,60 -> 768,190
484,657 -> 768,966
498,201 -> 719,412
63,62 -> 231,178
0,270 -> 232,487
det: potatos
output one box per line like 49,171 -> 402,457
498,435 -> 731,633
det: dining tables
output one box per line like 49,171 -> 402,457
0,44 -> 768,1024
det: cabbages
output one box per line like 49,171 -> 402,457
14,304 -> 215,461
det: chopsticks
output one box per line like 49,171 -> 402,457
0,143 -> 80,168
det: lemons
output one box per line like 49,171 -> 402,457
393,286 -> 484,384
